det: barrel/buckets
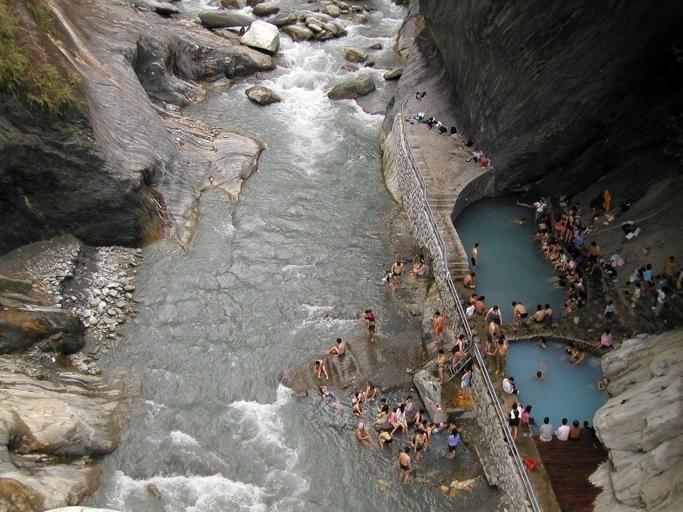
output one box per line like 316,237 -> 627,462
527,457 -> 537,471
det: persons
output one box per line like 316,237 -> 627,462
462,271 -> 476,289
318,385 -> 342,411
348,384 -> 459,487
409,110 -> 490,170
408,254 -> 426,276
313,358 -> 329,381
431,293 -> 508,400
320,337 -> 346,357
470,240 -> 478,273
362,309 -> 377,342
507,186 -> 682,442
384,270 -> 390,286
414,88 -> 427,101
390,259 -> 403,290
363,309 -> 371,328
408,260 -> 427,279
